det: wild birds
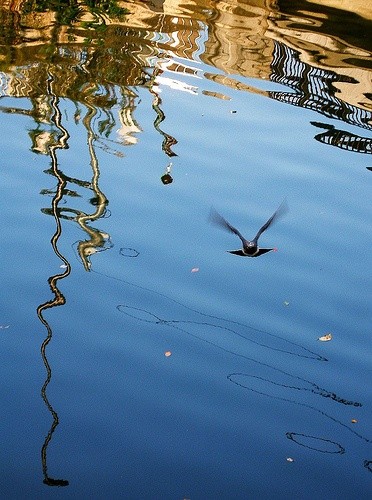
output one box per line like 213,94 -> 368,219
208,201 -> 286,258
